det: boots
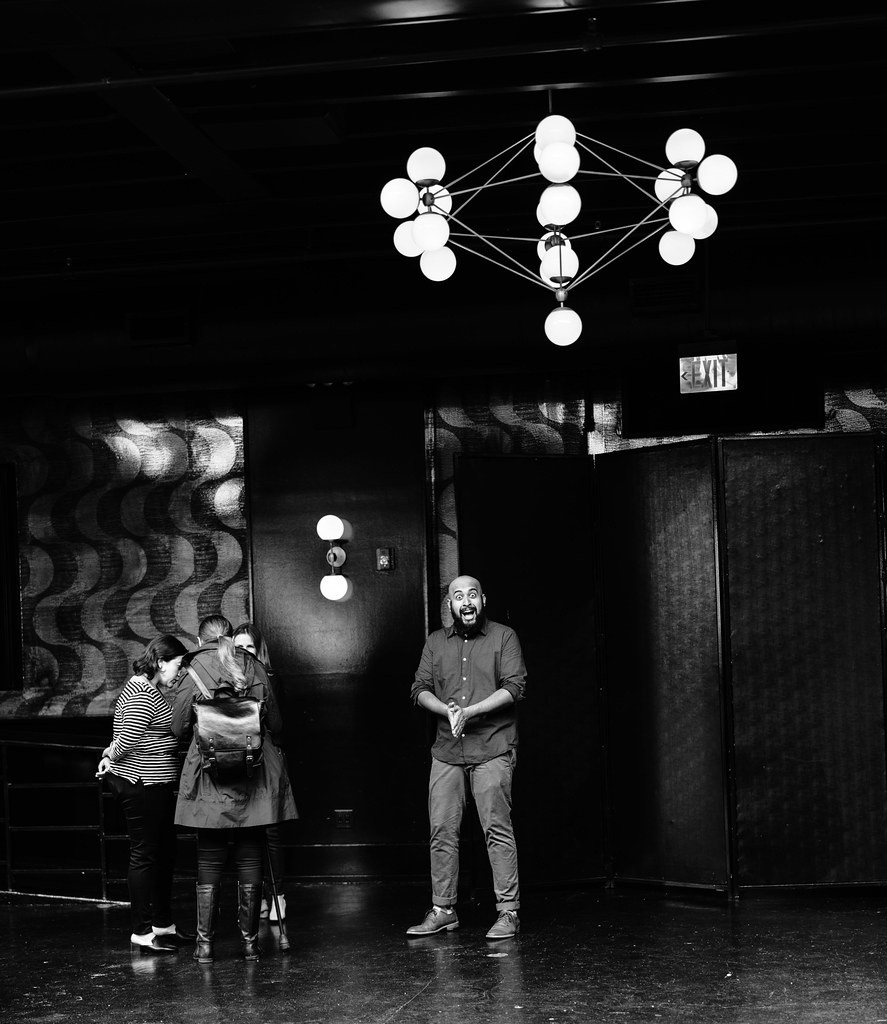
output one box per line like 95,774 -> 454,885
193,884 -> 219,963
236,881 -> 264,959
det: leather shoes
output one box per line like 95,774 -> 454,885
406,909 -> 459,937
157,929 -> 196,942
485,910 -> 519,939
140,939 -> 177,953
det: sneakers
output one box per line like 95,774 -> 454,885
270,895 -> 287,920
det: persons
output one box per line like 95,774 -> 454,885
231,623 -> 289,923
94,635 -> 195,958
406,575 -> 530,943
169,614 -> 282,965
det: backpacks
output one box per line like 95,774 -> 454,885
184,654 -> 264,773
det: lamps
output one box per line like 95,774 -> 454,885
378,84 -> 743,345
314,514 -> 351,600
